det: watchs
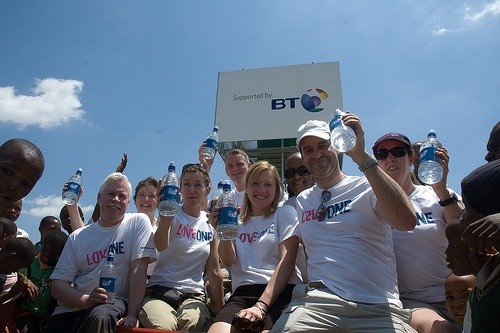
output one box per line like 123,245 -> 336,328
439,192 -> 459,207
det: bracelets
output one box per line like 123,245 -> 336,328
358,154 -> 380,172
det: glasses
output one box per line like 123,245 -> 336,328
315,187 -> 331,221
373,146 -> 408,160
284,166 -> 312,179
181,163 -> 207,173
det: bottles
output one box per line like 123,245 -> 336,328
61,168 -> 83,206
329,109 -> 356,153
418,129 -> 443,184
159,161 -> 178,216
210,179 -> 223,215
215,179 -> 238,240
99,252 -> 116,304
202,126 -> 218,159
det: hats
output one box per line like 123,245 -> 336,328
372,130 -> 412,151
296,123 -> 331,148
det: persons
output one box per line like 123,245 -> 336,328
410,141 -> 432,185
0,198 -> 39,313
445,121 -> 500,333
138,164 -> 226,333
61,176 -> 159,284
16,230 -> 68,318
372,132 -> 465,333
42,172 -> 157,333
207,160 -> 299,333
26,216 -> 60,245
61,154 -> 129,235
199,143 -> 252,214
0,139 -> 45,207
283,150 -> 315,284
271,112 -> 420,333
